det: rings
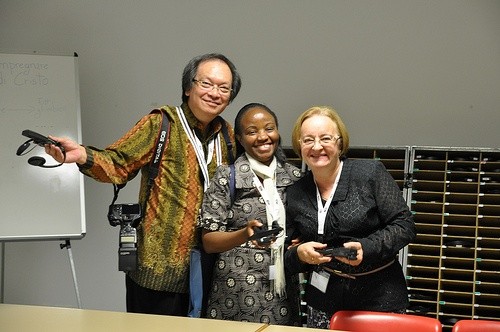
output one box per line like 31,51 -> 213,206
311,257 -> 314,261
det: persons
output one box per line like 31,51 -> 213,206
195,103 -> 305,324
44,53 -> 243,317
284,106 -> 417,329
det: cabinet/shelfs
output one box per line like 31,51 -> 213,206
281,146 -> 500,332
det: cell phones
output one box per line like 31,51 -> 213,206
247,226 -> 283,241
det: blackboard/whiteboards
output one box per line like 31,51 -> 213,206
0,50 -> 85,238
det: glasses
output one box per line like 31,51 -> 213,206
193,79 -> 232,94
299,134 -> 341,146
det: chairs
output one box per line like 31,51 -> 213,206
452,320 -> 500,332
330,311 -> 442,332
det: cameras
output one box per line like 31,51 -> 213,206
107,202 -> 143,272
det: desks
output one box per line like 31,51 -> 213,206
0,303 -> 268,332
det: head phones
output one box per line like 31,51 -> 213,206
16,139 -> 66,168
